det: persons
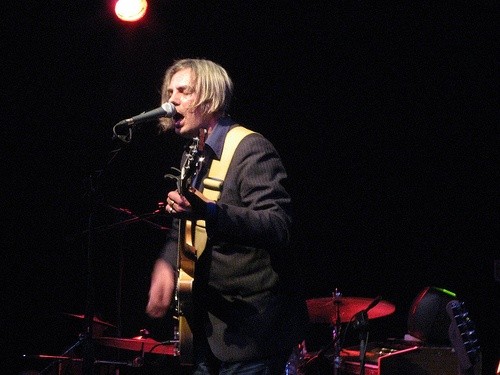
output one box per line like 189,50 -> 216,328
146,59 -> 310,375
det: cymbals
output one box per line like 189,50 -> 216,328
60,313 -> 117,328
89,336 -> 177,354
305,297 -> 395,323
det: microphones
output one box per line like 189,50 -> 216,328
116,102 -> 176,128
365,296 -> 384,313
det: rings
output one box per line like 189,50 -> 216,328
169,207 -> 173,214
170,200 -> 175,206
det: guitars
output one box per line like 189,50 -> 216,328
173,129 -> 208,359
